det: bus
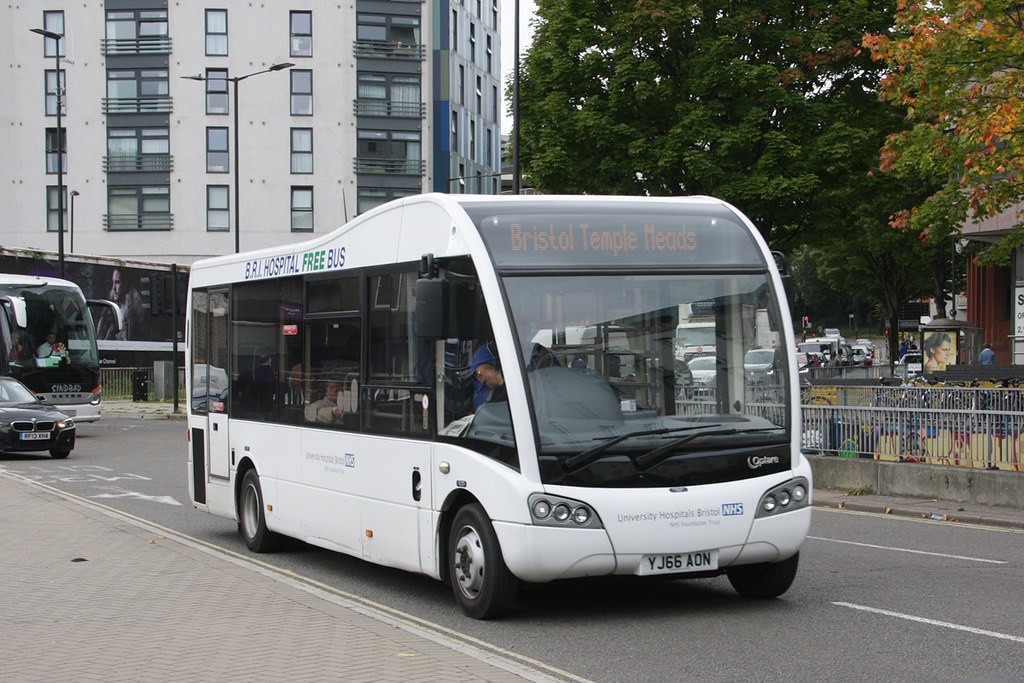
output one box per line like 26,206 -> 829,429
185,192 -> 813,619
0,273 -> 123,423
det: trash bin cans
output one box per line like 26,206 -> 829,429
132,371 -> 148,401
832,421 -> 876,459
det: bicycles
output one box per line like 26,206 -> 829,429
856,373 -> 1023,427
756,375 -> 834,422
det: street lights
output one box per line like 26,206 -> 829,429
29,28 -> 64,279
447,173 -> 502,193
180,62 -> 296,253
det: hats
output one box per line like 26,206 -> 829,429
982,343 -> 990,348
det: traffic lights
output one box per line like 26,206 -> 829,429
140,273 -> 159,316
802,316 -> 808,328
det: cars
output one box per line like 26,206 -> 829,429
674,323 -> 875,395
894,353 -> 922,380
0,376 -> 76,458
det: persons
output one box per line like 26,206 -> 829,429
977,343 -> 996,365
9,332 -> 24,360
96,266 -> 150,342
898,335 -> 918,363
471,301 -> 563,411
923,332 -> 951,374
816,324 -> 823,336
254,348 -> 301,404
307,380 -> 350,426
37,332 -> 61,357
